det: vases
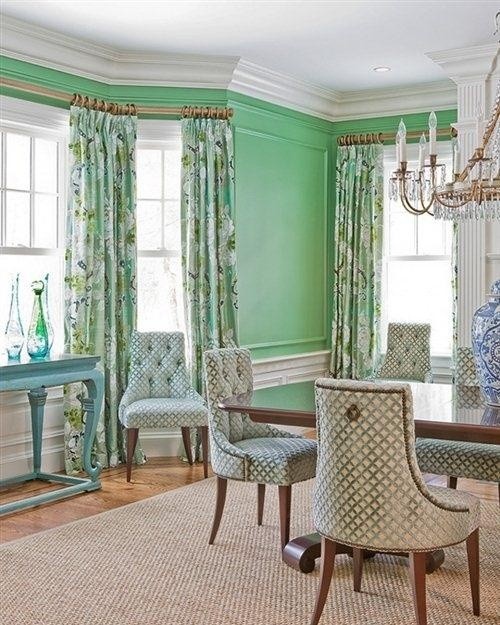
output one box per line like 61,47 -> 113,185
4,272 -> 54,361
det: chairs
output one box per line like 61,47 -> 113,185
202,348 -> 317,561
415,347 -> 500,508
359,322 -> 433,384
119,331 -> 210,481
311,378 -> 481,625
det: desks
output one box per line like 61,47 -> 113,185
0,353 -> 105,519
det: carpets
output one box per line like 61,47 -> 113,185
0,475 -> 500,625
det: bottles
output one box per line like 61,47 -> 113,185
4,271 -> 54,358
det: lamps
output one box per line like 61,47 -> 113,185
388,11 -> 500,223
